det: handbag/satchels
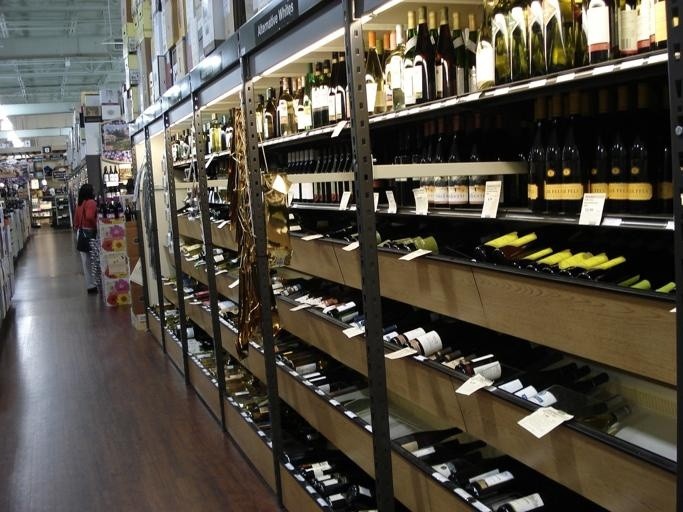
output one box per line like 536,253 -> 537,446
76,229 -> 96,251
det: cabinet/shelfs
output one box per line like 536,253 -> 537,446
0,149 -> 67,228
129,1 -> 682,511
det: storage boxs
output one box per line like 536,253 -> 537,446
96,213 -> 147,331
67,1 -> 277,171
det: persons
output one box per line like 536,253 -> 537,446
73,184 -> 98,294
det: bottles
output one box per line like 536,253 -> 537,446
96,165 -> 137,222
146,223 -> 675,512
169,0 -> 675,219
